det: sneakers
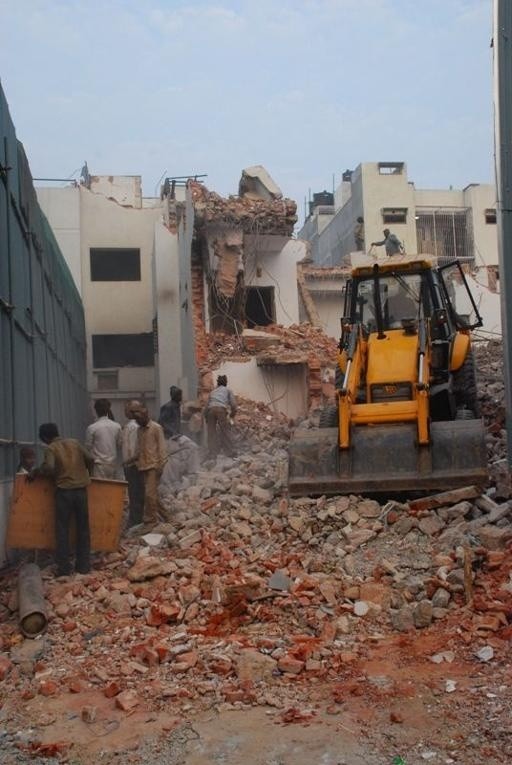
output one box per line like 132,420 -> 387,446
136,519 -> 160,535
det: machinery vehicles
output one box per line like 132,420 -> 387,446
282,256 -> 494,506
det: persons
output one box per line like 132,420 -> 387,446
371,229 -> 401,258
383,283 -> 417,320
15,386 -> 199,577
201,375 -> 237,465
354,217 -> 364,251
